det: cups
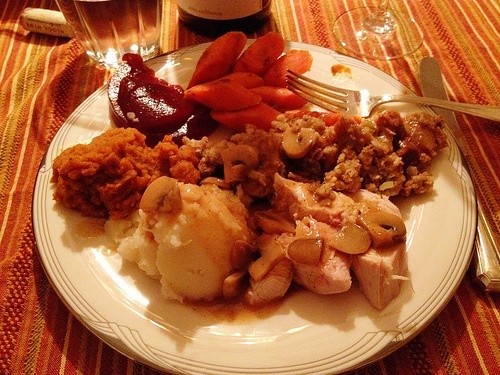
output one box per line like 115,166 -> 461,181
55,0 -> 162,76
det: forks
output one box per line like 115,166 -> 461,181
283,68 -> 500,123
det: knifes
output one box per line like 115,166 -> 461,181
418,56 -> 500,293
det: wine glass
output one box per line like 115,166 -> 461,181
332,0 -> 423,61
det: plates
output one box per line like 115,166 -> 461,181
34,38 -> 476,375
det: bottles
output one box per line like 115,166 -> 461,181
176,0 -> 272,38
